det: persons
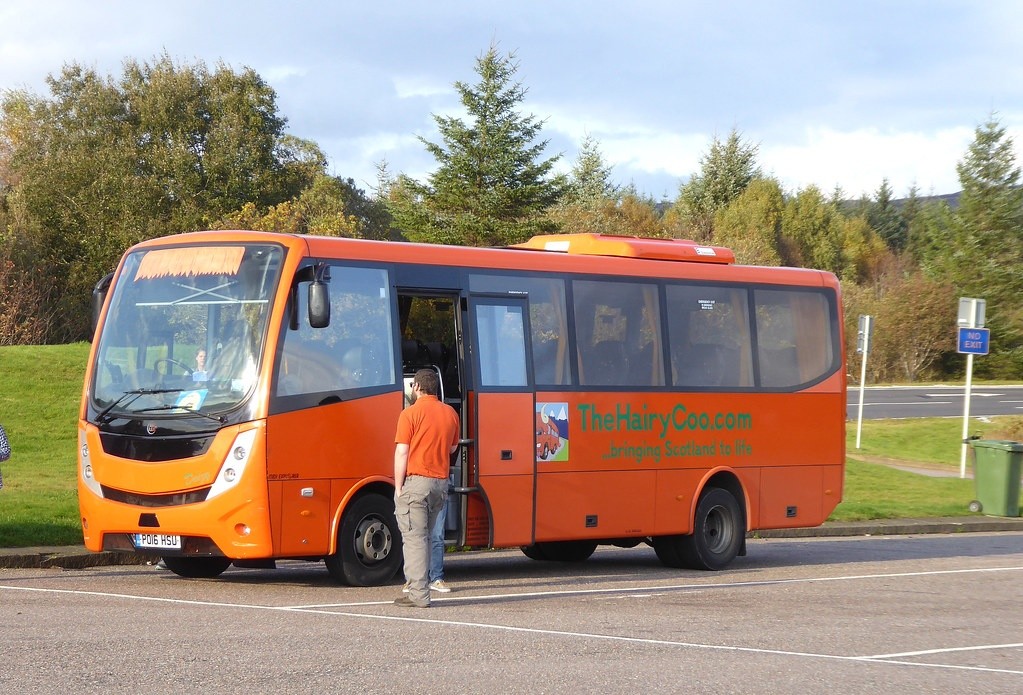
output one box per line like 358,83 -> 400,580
184,348 -> 206,378
393,367 -> 459,608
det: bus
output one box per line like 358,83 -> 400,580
78,232 -> 848,588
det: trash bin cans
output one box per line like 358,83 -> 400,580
961,431 -> 1023,518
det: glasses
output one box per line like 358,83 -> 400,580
410,382 -> 419,387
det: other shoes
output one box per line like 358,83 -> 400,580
428,579 -> 451,593
402,583 -> 409,593
394,596 -> 430,607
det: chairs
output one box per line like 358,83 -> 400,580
400,339 -> 458,398
540,338 -> 802,389
